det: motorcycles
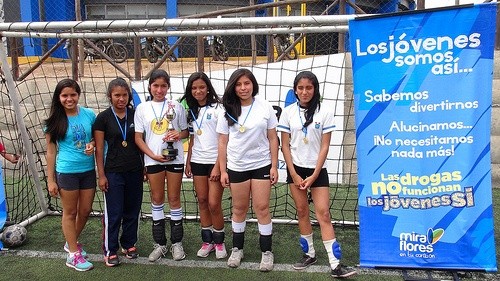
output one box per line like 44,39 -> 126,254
268,25 -> 298,61
139,27 -> 178,63
62,28 -> 128,63
203,34 -> 229,61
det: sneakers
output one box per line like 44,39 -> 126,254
121,246 -> 139,259
259,250 -> 274,272
148,244 -> 168,262
65,253 -> 93,271
104,254 -> 121,266
228,246 -> 244,268
169,242 -> 185,261
197,242 -> 215,257
214,241 -> 227,259
331,263 -> 356,277
64,241 -> 86,258
292,253 -> 317,269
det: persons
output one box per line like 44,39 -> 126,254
184,72 -> 227,260
216,69 -> 278,272
44,79 -> 97,271
94,78 -> 144,266
278,71 -> 358,277
134,70 -> 189,261
0,144 -> 20,163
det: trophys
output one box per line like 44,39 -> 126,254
162,108 -> 178,157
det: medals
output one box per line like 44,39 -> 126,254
303,137 -> 308,142
157,126 -> 161,130
122,141 -> 127,147
197,130 -> 201,135
240,127 -> 245,132
77,142 -> 82,147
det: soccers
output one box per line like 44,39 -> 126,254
1,223 -> 28,248
